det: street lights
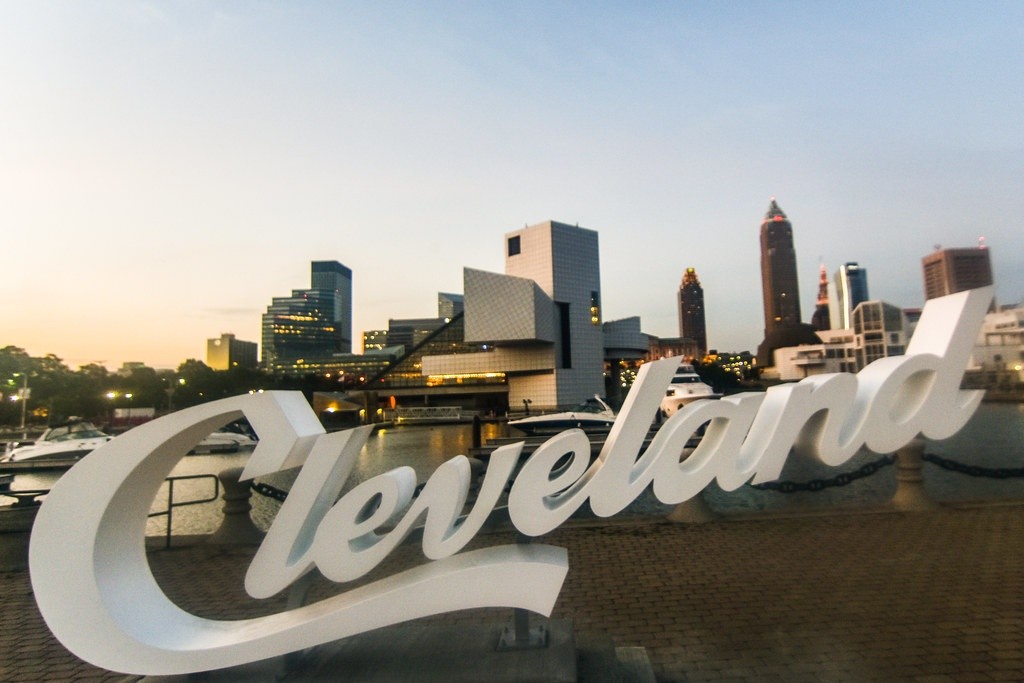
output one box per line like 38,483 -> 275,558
14,371 -> 28,429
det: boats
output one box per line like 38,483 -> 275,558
185,432 -> 258,454
654,361 -> 725,426
0,419 -> 116,469
509,393 -> 614,434
